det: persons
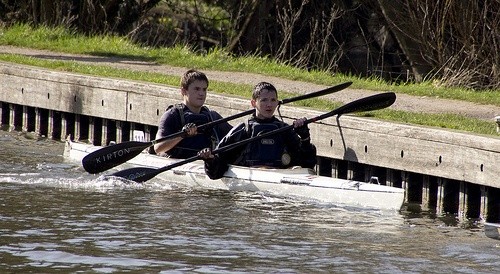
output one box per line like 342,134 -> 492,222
196,81 -> 317,180
153,70 -> 234,160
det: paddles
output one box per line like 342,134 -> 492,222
82,81 -> 353,175
103,91 -> 397,189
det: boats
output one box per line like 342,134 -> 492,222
62,132 -> 407,214
